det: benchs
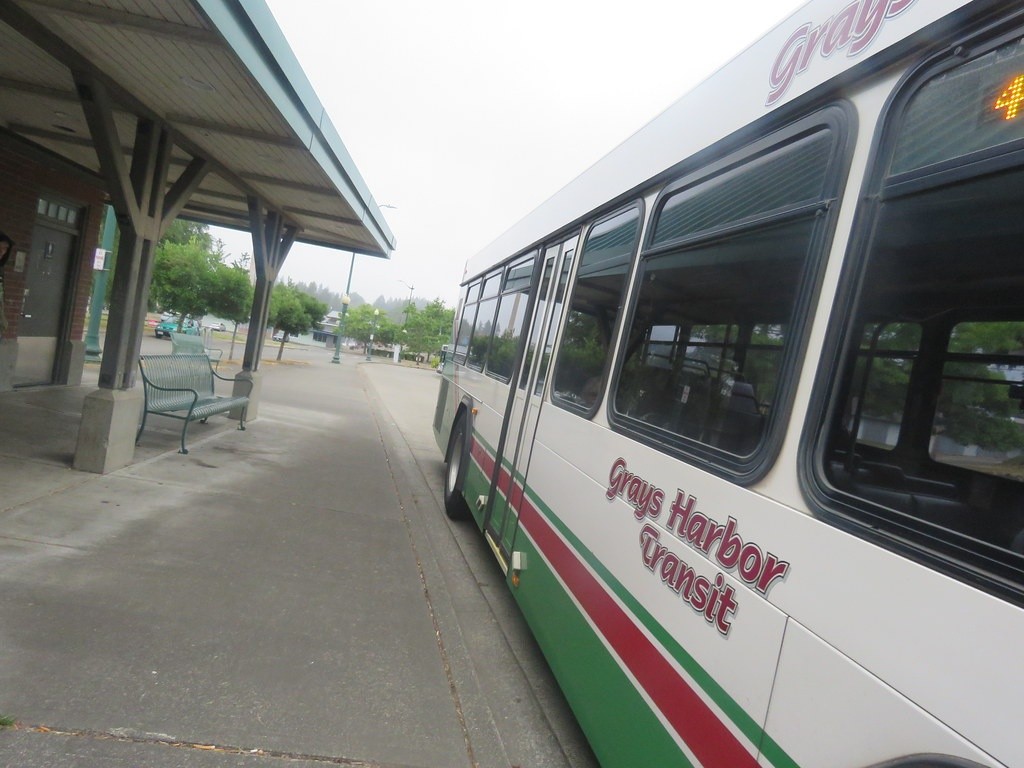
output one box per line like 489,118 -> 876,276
135,353 -> 254,455
170,331 -> 223,373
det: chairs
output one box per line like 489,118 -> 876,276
621,353 -> 764,459
826,448 -> 968,529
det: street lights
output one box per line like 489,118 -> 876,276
399,279 -> 414,361
331,204 -> 397,365
366,309 -> 379,360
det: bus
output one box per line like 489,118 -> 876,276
436,345 -> 447,374
429,0 -> 1024,768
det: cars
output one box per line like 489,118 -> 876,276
161,310 -> 176,321
155,316 -> 200,338
209,322 -> 226,332
273,332 -> 289,342
